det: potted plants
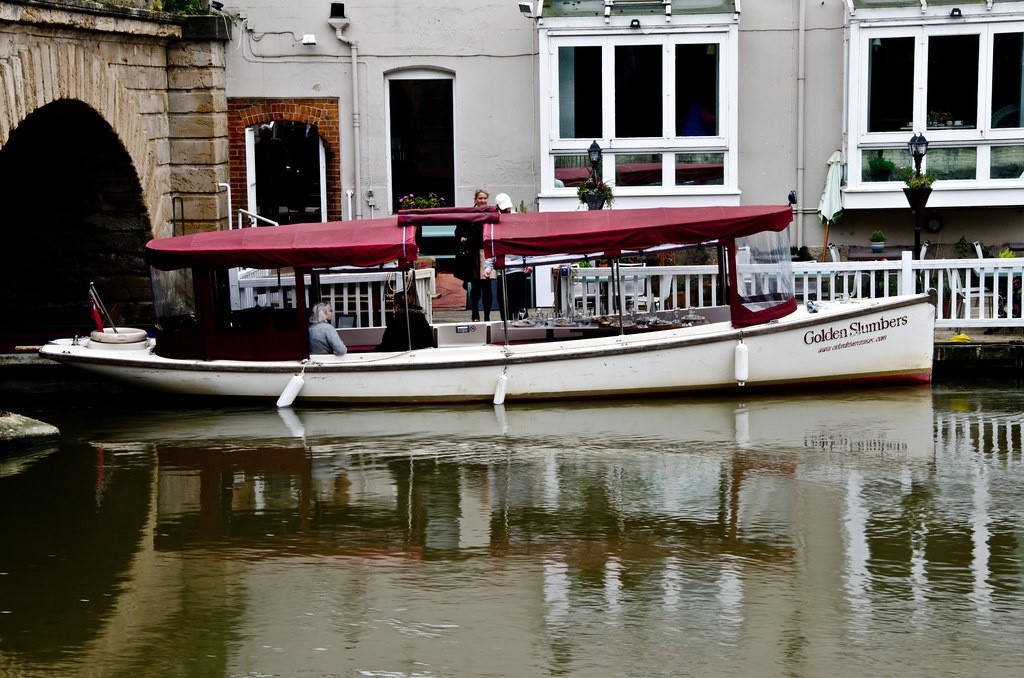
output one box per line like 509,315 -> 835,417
932,275 -> 961,318
902,172 -> 937,210
868,231 -> 888,253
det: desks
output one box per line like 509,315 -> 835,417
574,276 -> 648,315
761,271 -> 839,300
845,256 -> 902,296
973,263 -> 1022,335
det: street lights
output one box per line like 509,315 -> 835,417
900,133 -> 935,261
582,140 -> 604,269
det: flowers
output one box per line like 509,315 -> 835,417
398,193 -> 445,209
576,172 -> 616,210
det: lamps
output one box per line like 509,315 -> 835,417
517,2 -> 534,14
301,33 -> 317,46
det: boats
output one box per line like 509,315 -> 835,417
37,203 -> 937,406
71,381 -> 937,468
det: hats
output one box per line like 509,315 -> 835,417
495,193 -> 512,210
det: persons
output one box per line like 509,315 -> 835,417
455,190 -> 497,322
309,302 -> 347,355
372,291 -> 437,352
482,193 -> 533,320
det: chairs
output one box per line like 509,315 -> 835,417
777,260 -> 817,301
889,241 -> 929,293
956,270 -> 1005,337
835,271 -> 857,298
725,246 -> 752,286
828,244 -> 870,279
942,257 -> 991,331
973,241 -> 992,307
574,277 -> 603,316
632,276 -> 673,311
612,262 -> 646,312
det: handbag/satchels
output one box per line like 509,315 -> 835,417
454,242 -> 475,281
